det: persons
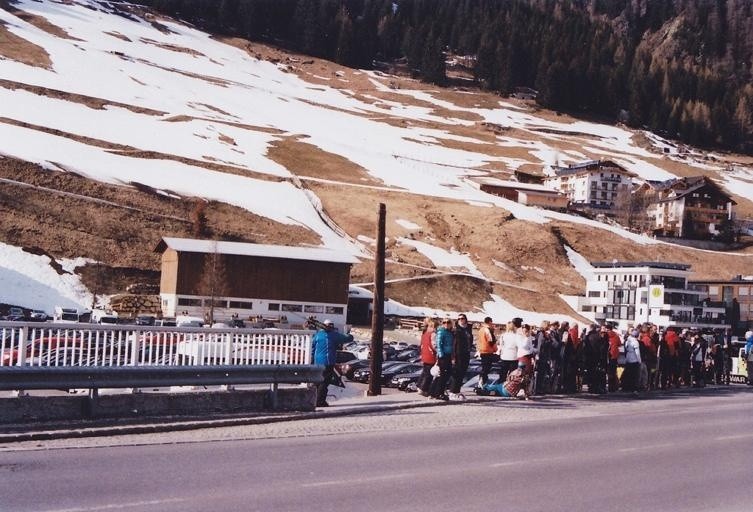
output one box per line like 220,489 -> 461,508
742,330 -> 752,385
417,312 -> 473,402
475,317 -> 732,397
310,320 -> 354,407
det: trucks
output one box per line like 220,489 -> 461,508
93,310 -> 119,325
52,305 -> 80,324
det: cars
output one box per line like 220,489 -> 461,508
465,346 -> 506,389
133,314 -> 154,325
728,348 -> 748,383
29,308 -> 48,322
7,306 -> 25,320
0,325 -> 426,394
